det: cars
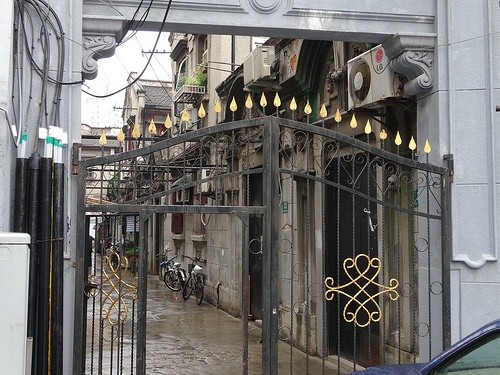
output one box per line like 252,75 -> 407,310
347,316 -> 499,375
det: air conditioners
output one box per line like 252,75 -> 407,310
346,44 -> 412,117
122,166 -> 131,180
196,169 -> 216,193
242,45 -> 277,87
179,107 -> 199,132
174,189 -> 189,203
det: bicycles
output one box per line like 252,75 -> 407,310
155,248 -> 174,286
180,253 -> 207,305
164,254 -> 188,296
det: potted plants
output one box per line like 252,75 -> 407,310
179,58 -> 208,94
